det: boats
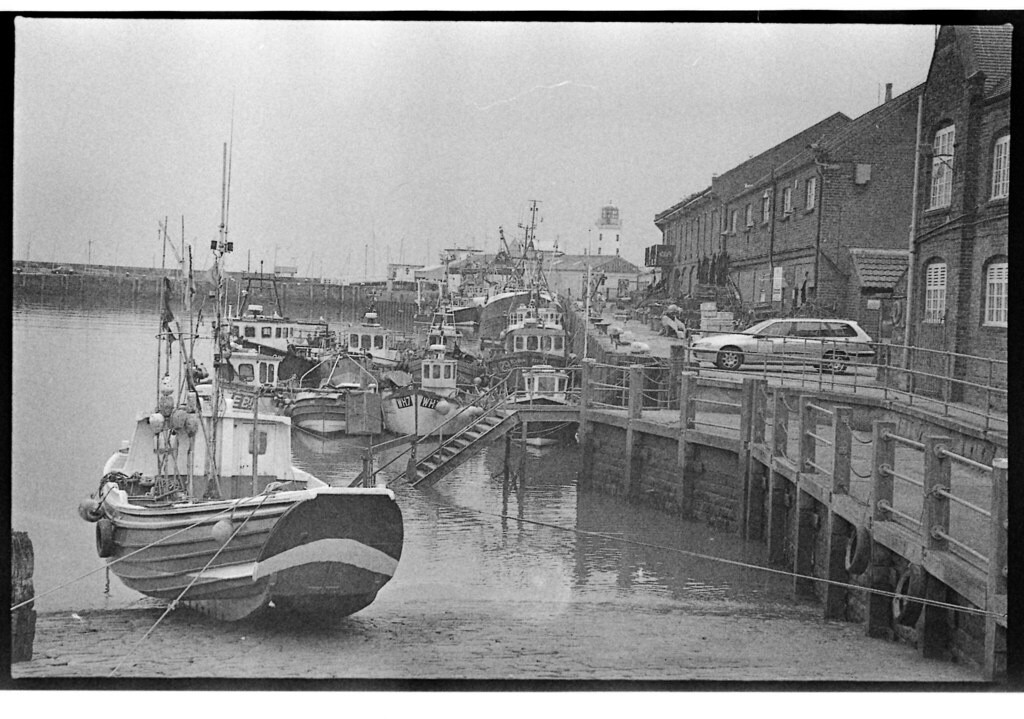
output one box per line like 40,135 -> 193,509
336,287 -> 415,378
380,341 -> 485,446
270,344 -> 381,439
415,198 -> 580,448
76,97 -> 405,627
186,278 -> 333,421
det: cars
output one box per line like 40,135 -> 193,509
691,318 -> 876,375
52,266 -> 73,275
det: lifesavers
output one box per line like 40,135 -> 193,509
844,525 -> 871,576
891,563 -> 928,626
94,518 -> 114,558
